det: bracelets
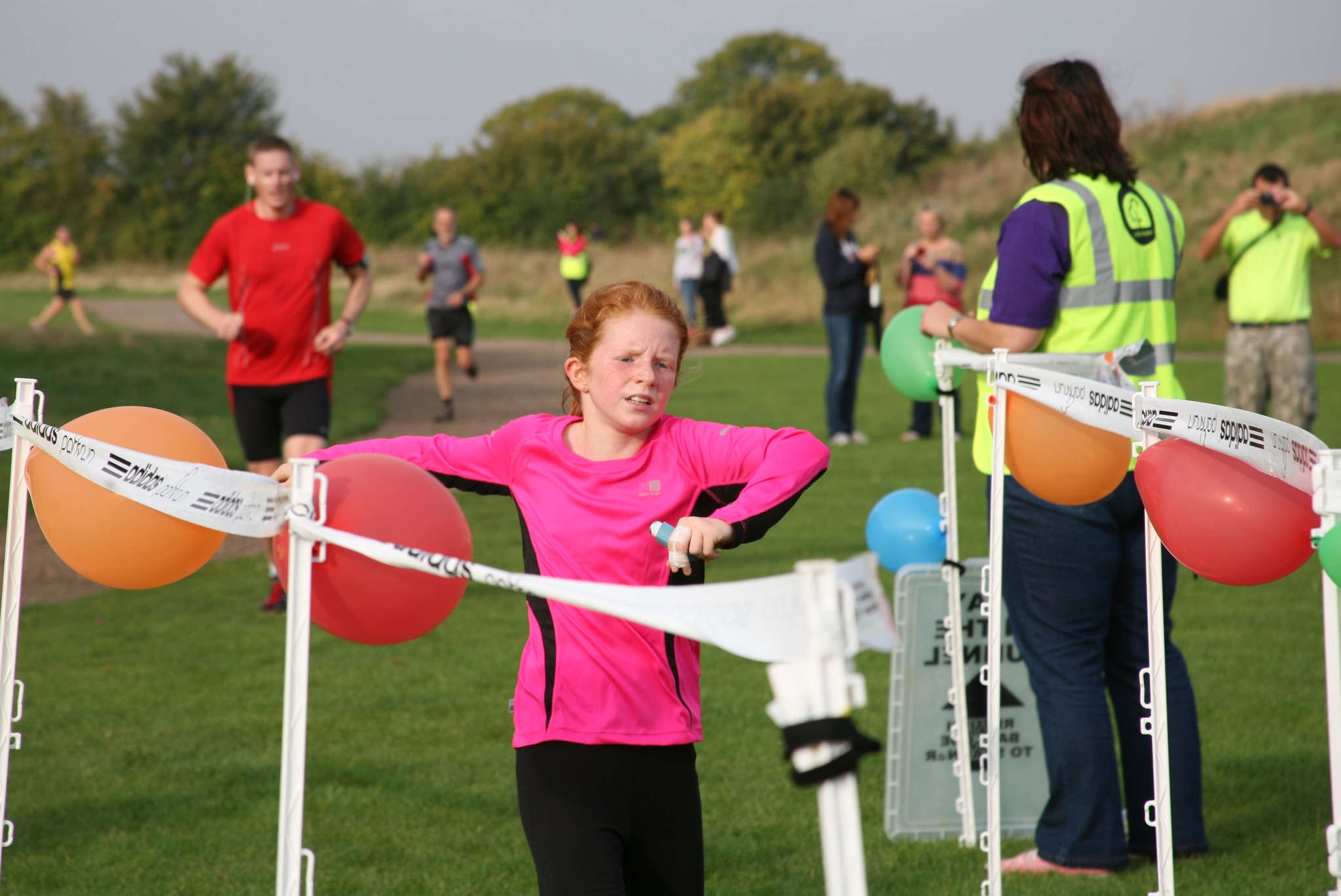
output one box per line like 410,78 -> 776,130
948,316 -> 963,340
461,289 -> 470,300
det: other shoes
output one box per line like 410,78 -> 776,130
433,403 -> 454,421
1000,847 -> 1108,877
852,430 -> 873,445
259,577 -> 285,614
830,432 -> 852,446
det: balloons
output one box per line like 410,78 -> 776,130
869,306 -> 1341,594
23,404 -> 470,642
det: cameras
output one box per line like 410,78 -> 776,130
1259,193 -> 1273,204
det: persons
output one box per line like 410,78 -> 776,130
27,227 -> 98,338
672,208 -> 739,348
918,62 -> 1209,878
264,281 -> 830,896
176,136 -> 376,611
813,188 -> 884,451
1196,162 -> 1341,434
556,221 -> 589,311
893,203 -> 968,443
415,206 -> 486,424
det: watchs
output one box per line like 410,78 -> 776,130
340,317 -> 353,337
1304,202 -> 1313,215
